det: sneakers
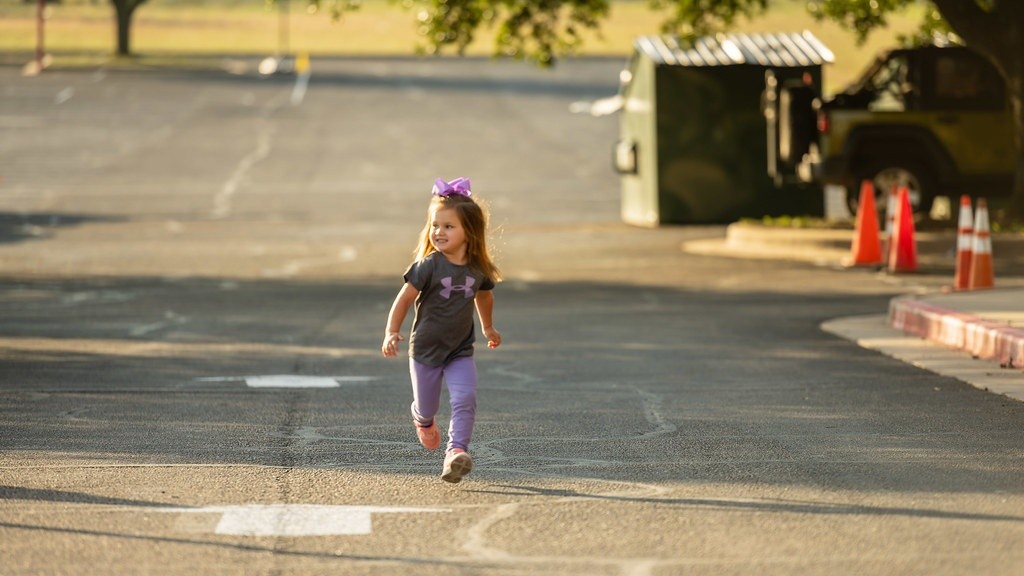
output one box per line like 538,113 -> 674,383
413,419 -> 440,448
440,449 -> 471,483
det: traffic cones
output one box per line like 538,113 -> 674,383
944,195 -> 974,291
888,184 -> 920,273
967,196 -> 994,291
884,183 -> 897,267
843,180 -> 883,267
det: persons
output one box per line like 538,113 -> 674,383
381,178 -> 502,484
